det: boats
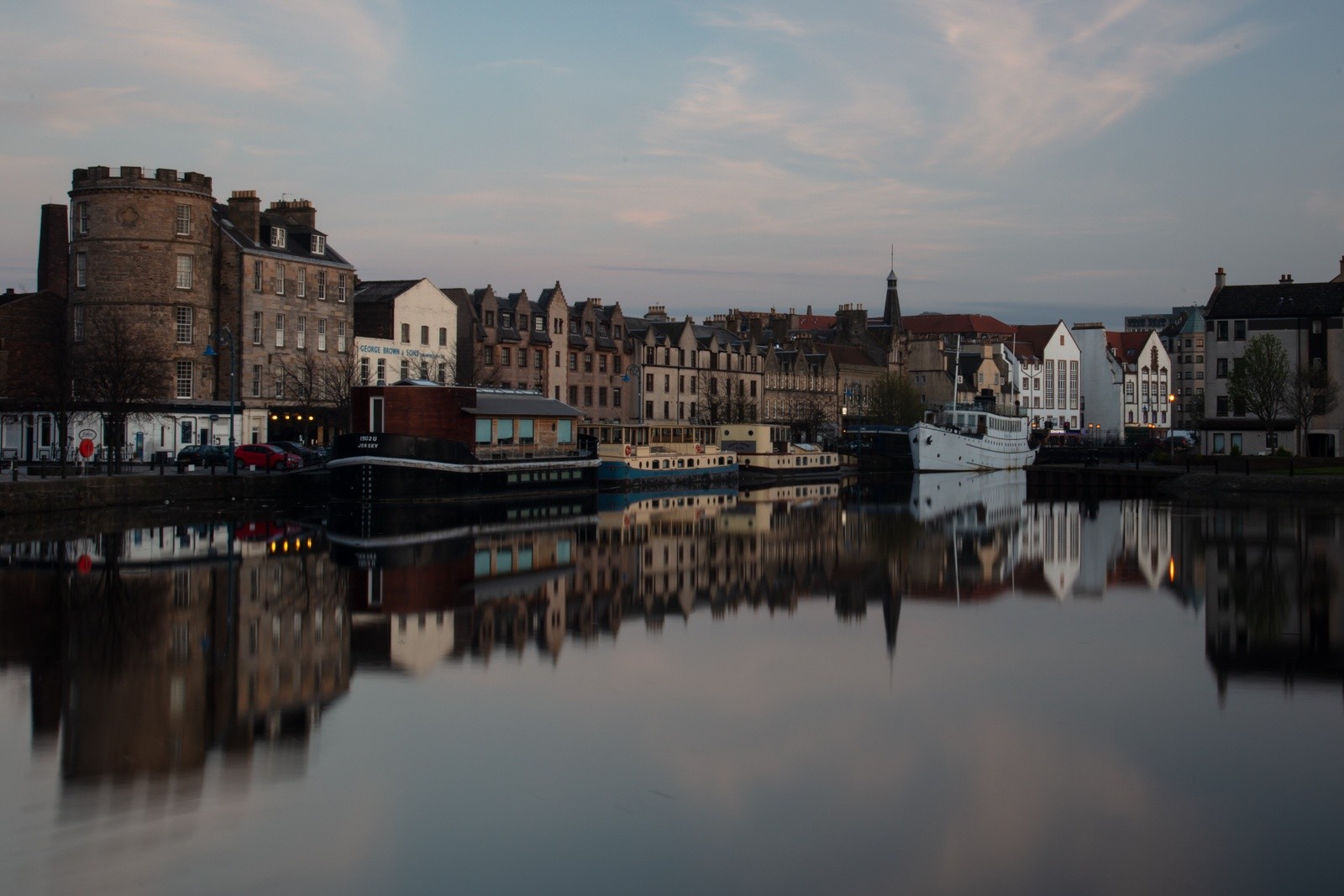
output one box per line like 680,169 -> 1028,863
714,475 -> 840,536
910,470 -> 1027,609
595,441 -> 740,489
319,489 -> 601,615
714,423 -> 840,477
907,334 -> 1042,473
597,488 -> 739,530
326,378 -> 605,504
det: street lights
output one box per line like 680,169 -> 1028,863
201,326 -> 235,473
1095,424 -> 1100,445
1169,394 -> 1175,457
1089,423 -> 1093,441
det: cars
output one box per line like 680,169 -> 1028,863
177,445 -> 229,467
269,440 -> 326,464
235,444 -> 304,471
234,519 -> 301,543
1134,436 -> 1189,451
